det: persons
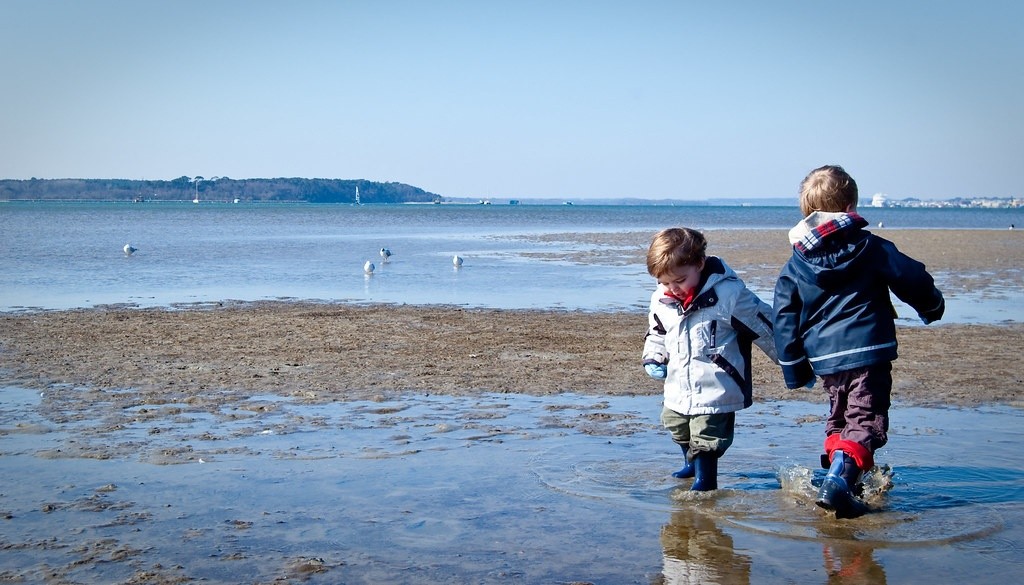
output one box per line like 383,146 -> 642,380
642,226 -> 772,491
773,166 -> 945,518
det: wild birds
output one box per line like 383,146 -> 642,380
379,247 -> 396,260
452,254 -> 464,266
365,260 -> 376,275
877,218 -> 1016,231
123,243 -> 139,257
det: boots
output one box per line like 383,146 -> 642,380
811,455 -> 831,487
691,451 -> 717,491
814,449 -> 866,516
672,443 -> 695,478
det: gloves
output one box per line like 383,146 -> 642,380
644,363 -> 666,380
